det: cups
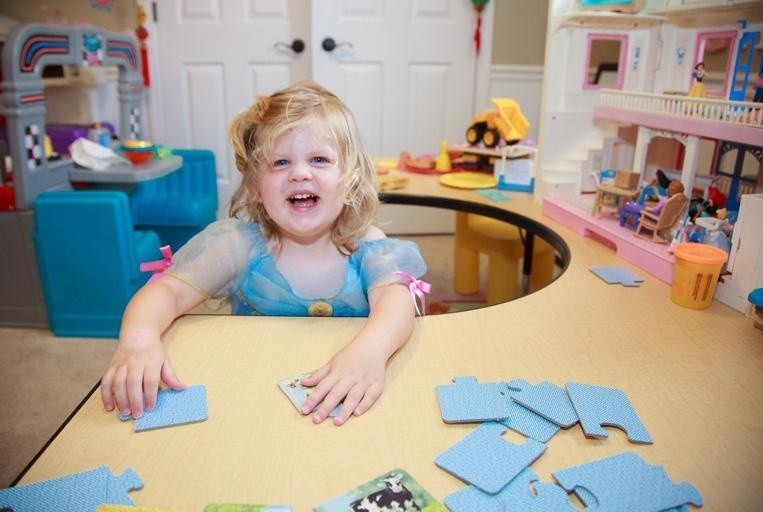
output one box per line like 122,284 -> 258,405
671,242 -> 728,311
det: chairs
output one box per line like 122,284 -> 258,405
30,149 -> 218,338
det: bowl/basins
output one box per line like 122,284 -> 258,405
120,139 -> 155,164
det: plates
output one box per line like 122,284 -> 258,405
439,171 -> 497,190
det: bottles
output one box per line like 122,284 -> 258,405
87,122 -> 110,149
435,140 -> 452,172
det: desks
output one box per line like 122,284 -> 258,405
4,159 -> 763,511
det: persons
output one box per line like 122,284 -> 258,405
685,62 -> 707,111
638,170 -> 733,253
98,77 -> 430,427
